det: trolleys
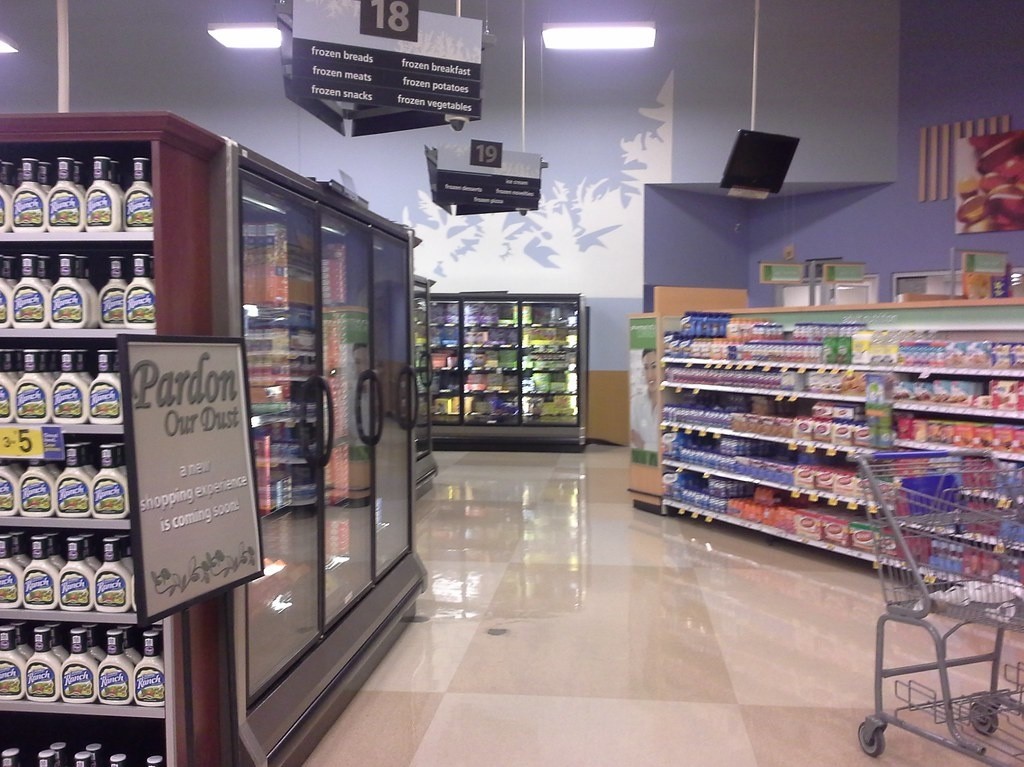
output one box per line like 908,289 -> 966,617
849,441 -> 1023,767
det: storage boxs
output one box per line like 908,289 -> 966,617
791,330 -> 1024,583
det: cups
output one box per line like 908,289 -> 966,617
1011,273 -> 1024,297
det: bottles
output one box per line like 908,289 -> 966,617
0,156 -> 153,233
0,349 -> 125,425
0,742 -> 165,767
665,364 -> 795,390
0,621 -> 165,707
679,311 -> 952,368
663,404 -> 793,439
664,477 -> 777,527
663,438 -> 794,485
0,531 -> 138,613
0,253 -> 158,329
929,540 -> 965,574
0,442 -> 130,519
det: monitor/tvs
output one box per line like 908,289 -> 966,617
719,129 -> 800,193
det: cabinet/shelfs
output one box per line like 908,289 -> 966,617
656,301 -> 1024,595
0,108 -> 226,766
